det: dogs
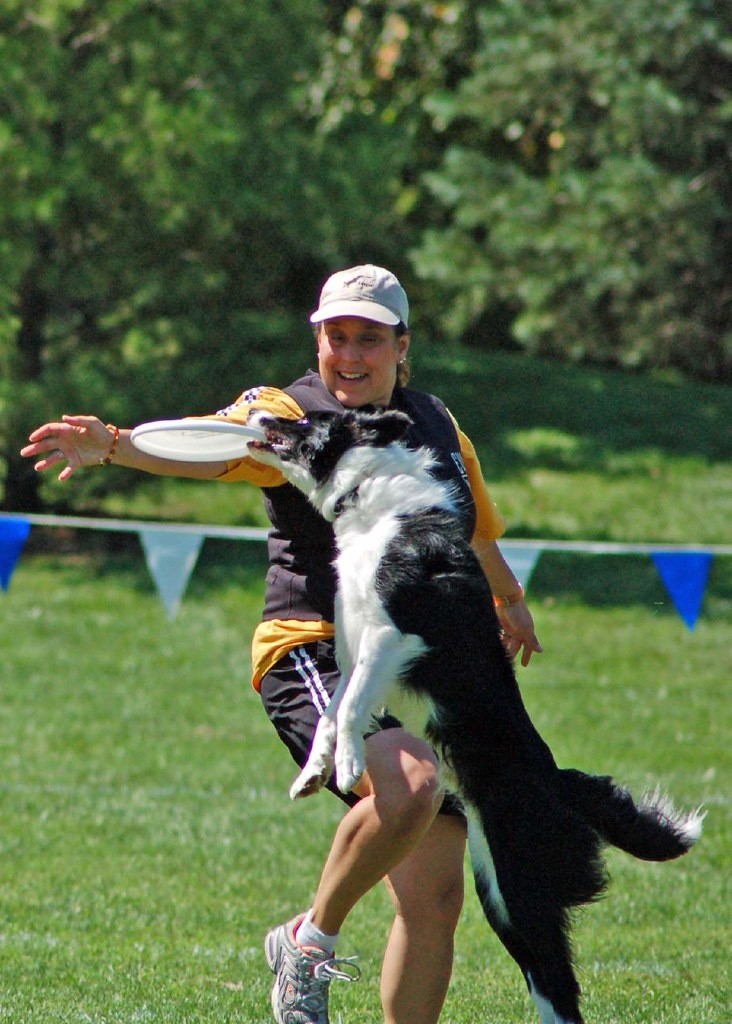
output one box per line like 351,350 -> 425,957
247,407 -> 709,1024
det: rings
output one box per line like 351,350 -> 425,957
55,450 -> 64,460
502,631 -> 511,637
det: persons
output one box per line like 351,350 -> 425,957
20,263 -> 544,1024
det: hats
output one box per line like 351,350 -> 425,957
311,264 -> 409,330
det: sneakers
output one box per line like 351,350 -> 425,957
265,914 -> 361,1024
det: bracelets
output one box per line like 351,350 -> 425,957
493,583 -> 525,606
100,423 -> 120,467
